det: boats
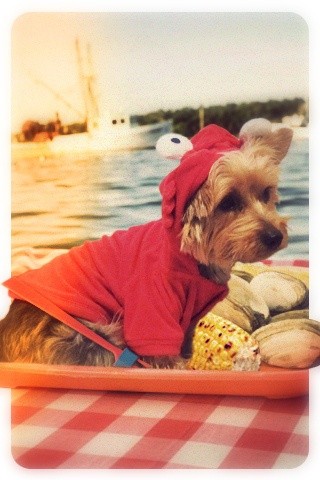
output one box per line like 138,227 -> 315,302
45,33 -> 174,153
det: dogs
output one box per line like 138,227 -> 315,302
0,126 -> 294,369
